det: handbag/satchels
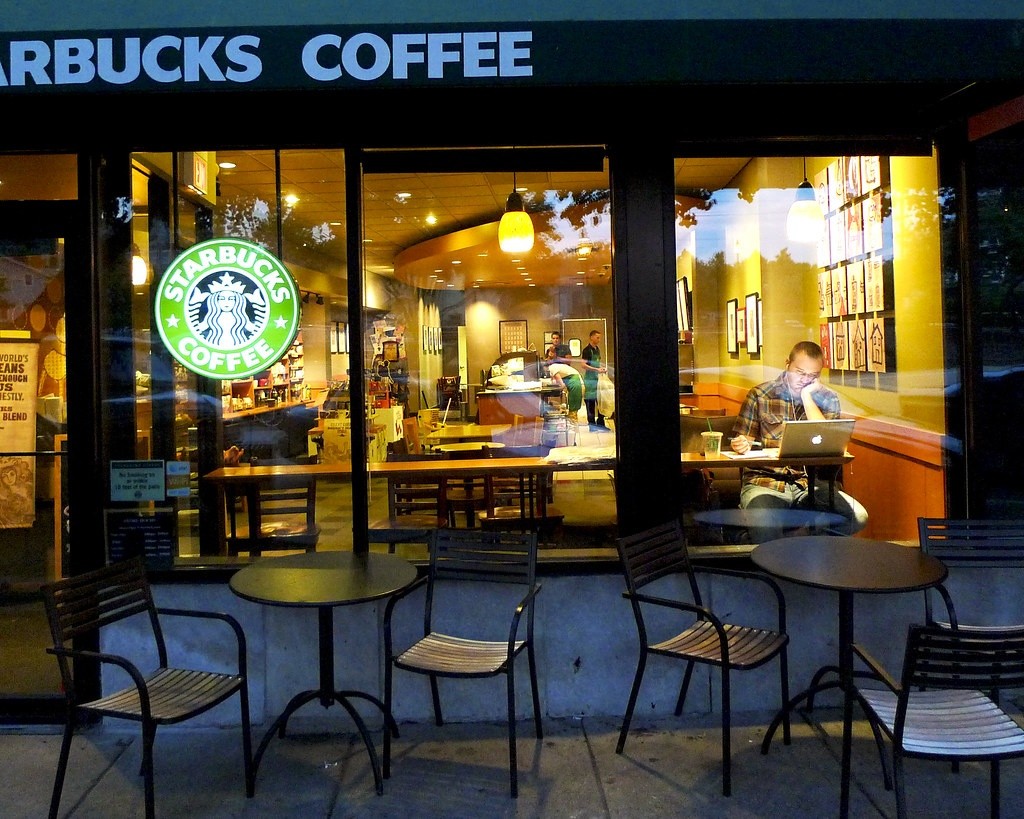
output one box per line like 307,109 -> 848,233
597,371 -> 615,417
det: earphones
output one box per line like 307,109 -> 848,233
786,364 -> 790,372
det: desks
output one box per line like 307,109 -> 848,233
203,424 -> 855,555
750,535 -> 948,790
227,551 -> 418,797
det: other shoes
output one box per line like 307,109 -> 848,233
589,420 -> 611,432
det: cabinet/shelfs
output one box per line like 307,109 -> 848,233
222,329 -> 305,414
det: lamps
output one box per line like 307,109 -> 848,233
785,157 -> 827,242
315,294 -> 324,305
302,293 -> 310,303
497,147 -> 534,254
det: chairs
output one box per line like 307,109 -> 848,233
225,454 -> 321,556
841,625 -> 1024,819
916,515 -> 1024,772
368,450 -> 449,554
387,438 -> 439,517
381,529 -> 544,800
614,515 -> 791,799
477,444 -> 565,548
37,555 -> 255,819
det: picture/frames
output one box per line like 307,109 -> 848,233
330,321 -> 338,353
745,291 -> 759,354
338,322 -> 346,353
727,298 -> 739,353
423,325 -> 442,350
736,307 -> 747,343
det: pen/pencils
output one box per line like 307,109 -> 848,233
727,437 -> 761,446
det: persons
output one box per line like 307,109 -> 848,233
731,341 -> 869,544
543,331 -> 611,433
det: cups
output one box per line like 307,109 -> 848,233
700,432 -> 723,461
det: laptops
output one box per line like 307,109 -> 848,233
763,419 -> 856,458
